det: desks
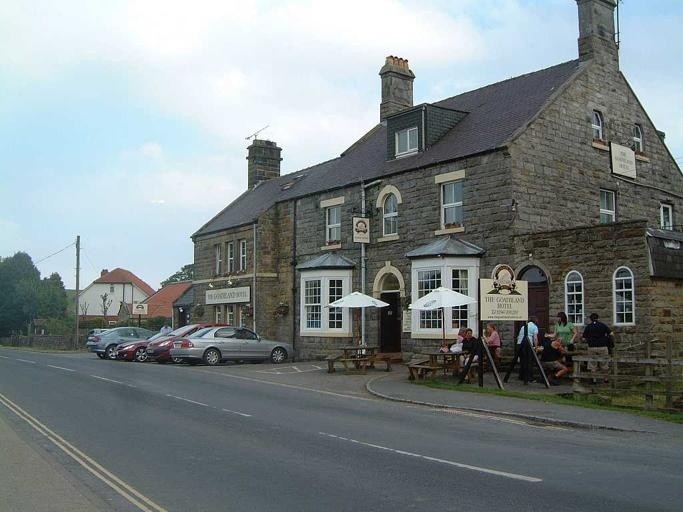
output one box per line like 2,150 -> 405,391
421,349 -> 469,377
338,345 -> 379,370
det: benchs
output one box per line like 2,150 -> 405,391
403,358 -> 462,381
324,353 -> 392,374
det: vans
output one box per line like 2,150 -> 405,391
146,322 -> 230,363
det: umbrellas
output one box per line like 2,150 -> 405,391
326,292 -> 390,369
408,287 -> 475,375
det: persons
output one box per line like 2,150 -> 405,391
160,322 -> 172,334
482,323 -> 500,372
544,311 -> 577,380
583,312 -> 613,385
516,317 -> 540,381
541,336 -> 569,385
439,329 -> 479,383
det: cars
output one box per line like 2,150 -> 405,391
86,326 -> 164,362
168,327 -> 292,366
